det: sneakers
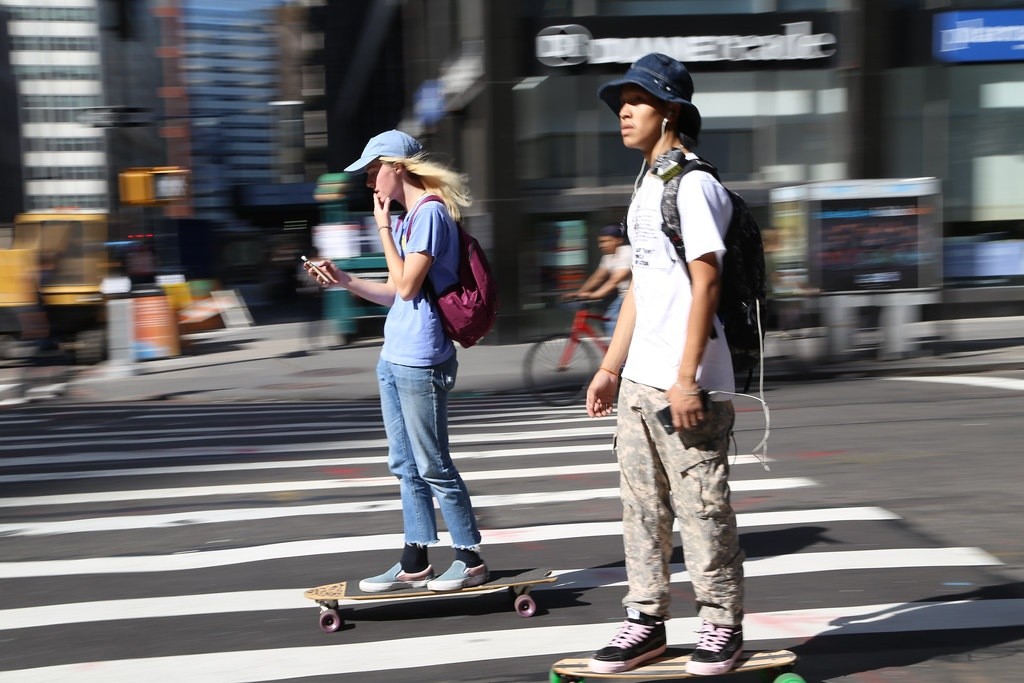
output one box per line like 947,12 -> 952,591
588,605 -> 667,673
684,621 -> 744,675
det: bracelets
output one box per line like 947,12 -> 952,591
598,367 -> 620,377
377,227 -> 393,233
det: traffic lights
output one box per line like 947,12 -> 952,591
152,171 -> 192,199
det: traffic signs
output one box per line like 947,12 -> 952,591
74,108 -> 155,126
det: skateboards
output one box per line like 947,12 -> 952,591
304,567 -> 559,634
548,649 -> 808,683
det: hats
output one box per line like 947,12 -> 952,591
598,52 -> 702,146
598,225 -> 624,237
343,128 -> 424,177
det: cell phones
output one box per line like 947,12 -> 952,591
301,255 -> 332,285
656,390 -> 715,435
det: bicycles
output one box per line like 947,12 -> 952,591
523,294 -> 617,409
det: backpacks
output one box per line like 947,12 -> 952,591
395,194 -> 498,349
652,148 -> 768,394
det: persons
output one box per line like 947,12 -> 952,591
586,54 -> 744,675
562,225 -> 632,369
304,129 -> 491,594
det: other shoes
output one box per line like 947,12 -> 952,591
358,561 -> 435,593
426,560 -> 489,590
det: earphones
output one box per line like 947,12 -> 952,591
663,110 -> 675,124
